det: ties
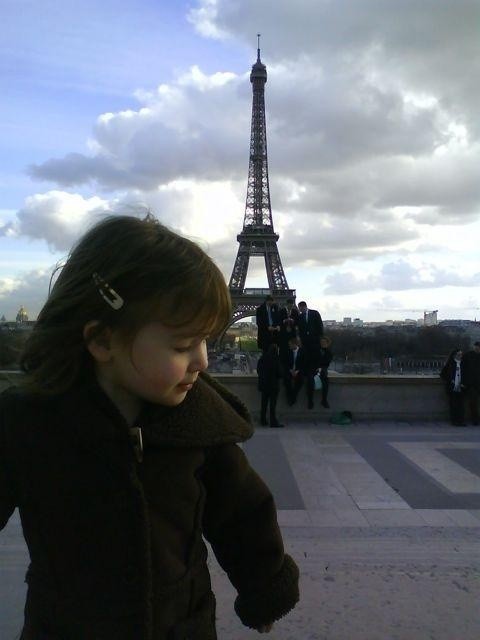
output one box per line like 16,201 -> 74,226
287,310 -> 290,318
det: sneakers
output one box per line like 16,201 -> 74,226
307,400 -> 314,410
260,420 -> 285,428
321,400 -> 331,409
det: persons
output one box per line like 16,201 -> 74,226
440,347 -> 468,428
253,292 -> 335,428
0,213 -> 301,638
462,340 -> 480,428
